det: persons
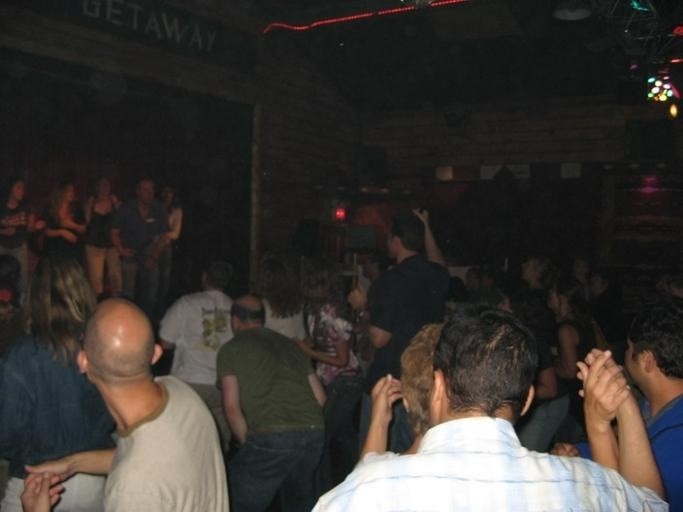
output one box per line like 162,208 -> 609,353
0,176 -> 43,312
410,208 -> 471,320
253,252 -> 326,410
457,241 -> 518,315
0,248 -> 112,512
559,228 -> 624,342
343,323 -> 445,480
20,294 -> 231,510
303,259 -> 364,503
538,272 -> 609,448
662,276 -> 683,304
36,181 -> 87,311
552,306 -> 683,511
156,183 -> 185,311
216,294 -> 325,512
366,211 -> 451,458
299,306 -> 669,512
495,235 -> 560,360
108,176 -> 167,300
81,180 -> 124,301
156,260 -> 239,456
